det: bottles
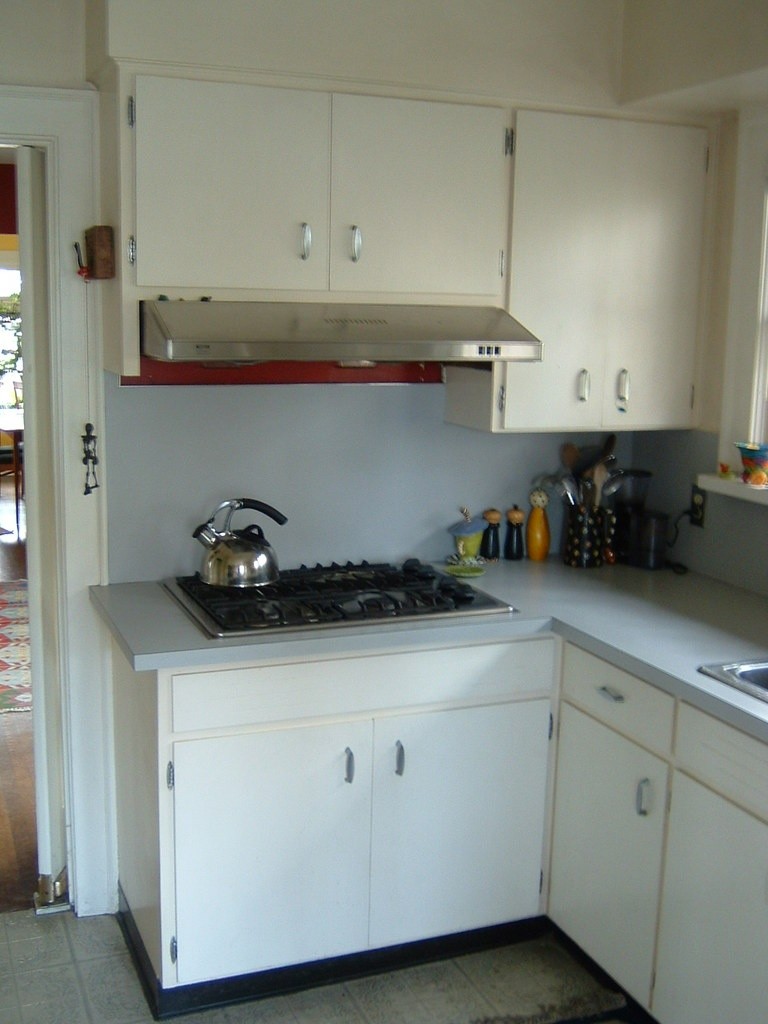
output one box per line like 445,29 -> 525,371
525,488 -> 551,561
504,504 -> 526,561
480,509 -> 502,561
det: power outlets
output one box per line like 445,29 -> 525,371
690,482 -> 706,529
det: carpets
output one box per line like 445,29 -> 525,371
0,578 -> 33,714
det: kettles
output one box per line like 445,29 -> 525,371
192,497 -> 288,590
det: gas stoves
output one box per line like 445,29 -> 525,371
159,558 -> 515,640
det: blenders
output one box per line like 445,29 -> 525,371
602,468 -> 654,567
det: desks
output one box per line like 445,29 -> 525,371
0,408 -> 24,528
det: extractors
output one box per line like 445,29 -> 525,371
141,299 -> 543,362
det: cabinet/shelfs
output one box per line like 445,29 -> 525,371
85,55 -> 511,378
441,102 -> 720,435
543,634 -> 768,1024
107,633 -> 558,1022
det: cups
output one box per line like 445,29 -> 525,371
562,503 -> 616,569
443,517 -> 489,577
640,511 -> 671,570
